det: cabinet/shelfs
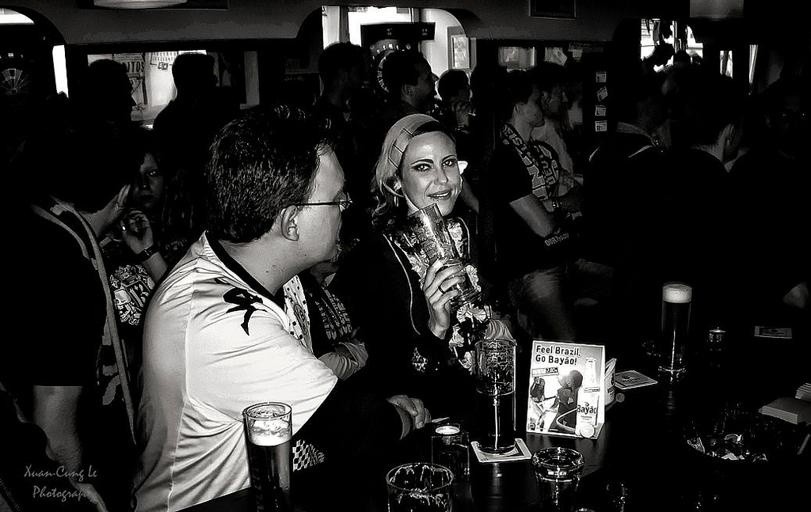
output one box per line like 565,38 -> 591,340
475,38 -> 570,73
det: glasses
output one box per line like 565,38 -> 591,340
296,192 -> 351,210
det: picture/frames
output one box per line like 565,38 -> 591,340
447,25 -> 471,69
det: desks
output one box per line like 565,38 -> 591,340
175,297 -> 811,512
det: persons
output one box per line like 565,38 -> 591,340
1,24 -> 811,511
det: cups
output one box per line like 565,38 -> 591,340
385,463 -> 453,512
431,420 -> 472,476
657,280 -> 691,375
705,322 -> 726,356
473,336 -> 518,456
241,402 -> 294,512
407,201 -> 482,309
533,447 -> 585,512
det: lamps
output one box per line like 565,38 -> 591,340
690,0 -> 745,22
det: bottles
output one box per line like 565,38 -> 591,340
576,358 -> 600,436
